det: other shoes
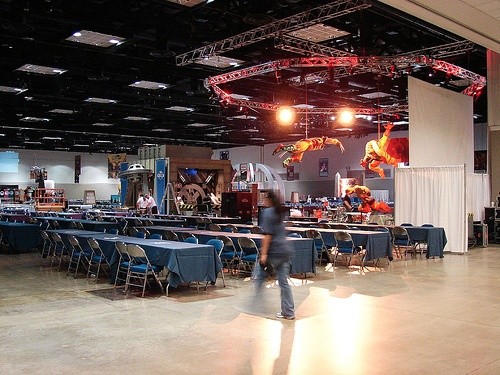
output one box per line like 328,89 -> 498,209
276,312 -> 295,319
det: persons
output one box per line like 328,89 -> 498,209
272,136 -> 345,168
360,123 -> 400,179
250,189 -> 295,319
344,190 -> 352,219
137,193 -> 156,208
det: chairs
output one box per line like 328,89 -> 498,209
0,205 -> 448,299
